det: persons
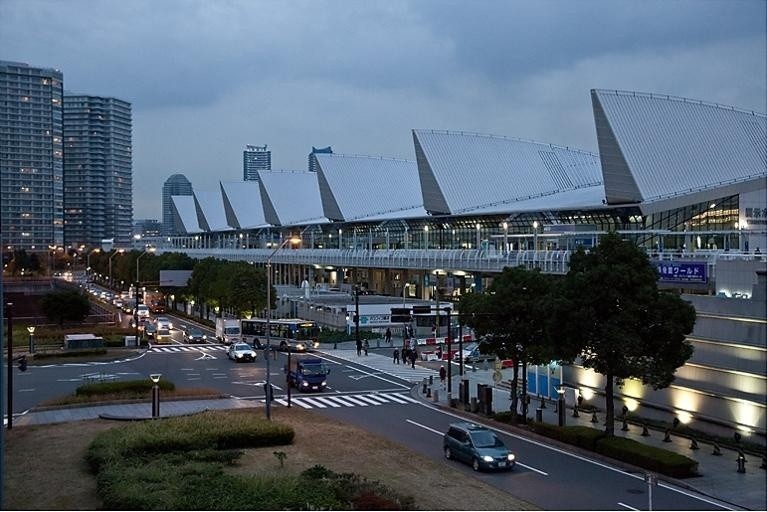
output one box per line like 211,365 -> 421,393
355,322 -> 446,390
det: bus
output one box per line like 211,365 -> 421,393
238,315 -> 323,353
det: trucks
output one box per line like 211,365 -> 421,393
285,351 -> 330,396
121,297 -> 136,314
215,315 -> 243,346
131,287 -> 167,313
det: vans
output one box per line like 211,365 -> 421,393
443,422 -> 518,472
452,341 -> 496,363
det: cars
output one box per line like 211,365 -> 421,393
89,288 -> 123,308
121,290 -> 130,299
132,303 -> 210,345
224,342 -> 261,363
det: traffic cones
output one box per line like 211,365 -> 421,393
572,404 -> 580,418
736,439 -> 747,461
621,419 -> 629,430
663,429 -> 671,442
641,422 -> 651,436
540,396 -> 546,408
760,450 -> 765,470
690,437 -> 699,450
712,437 -> 723,456
591,408 -> 598,423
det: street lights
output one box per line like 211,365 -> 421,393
402,283 -> 410,306
354,284 -> 364,338
26,325 -> 36,354
149,372 -> 162,418
86,248 -> 100,271
136,247 -> 156,283
264,235 -> 302,320
553,384 -> 570,428
107,248 -> 126,277
